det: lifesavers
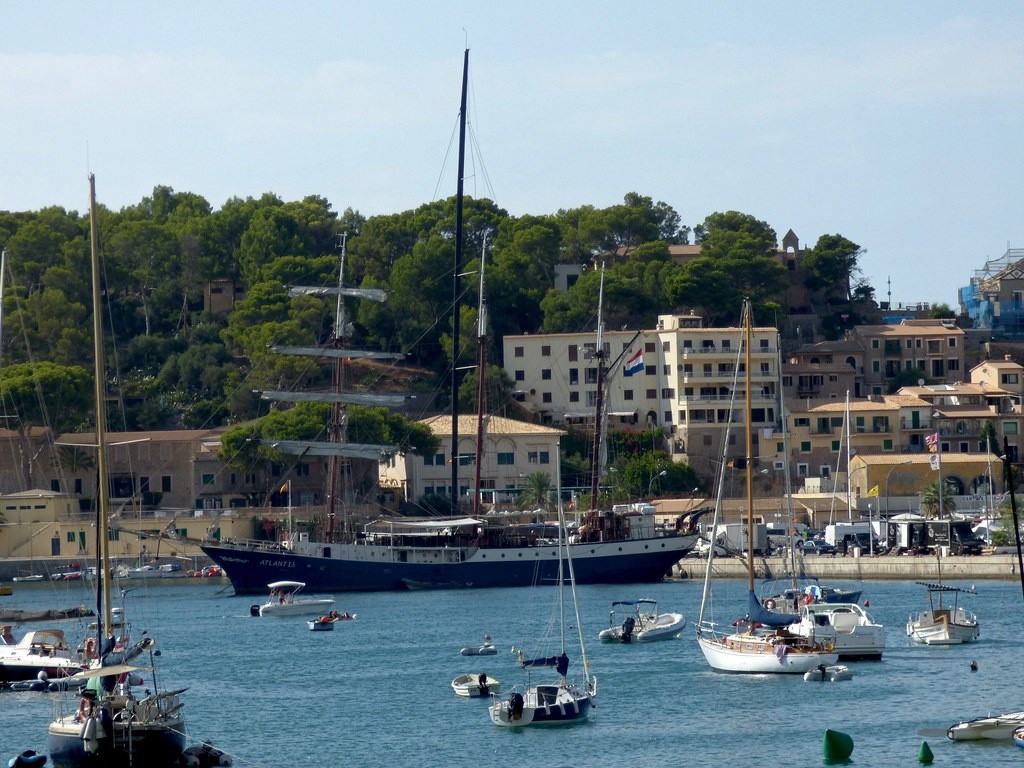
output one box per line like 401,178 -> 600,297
765,599 -> 777,611
530,529 -> 539,548
80,697 -> 93,723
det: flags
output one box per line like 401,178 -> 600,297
925,432 -> 938,446
624,347 -> 644,377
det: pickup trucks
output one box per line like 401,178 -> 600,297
837,533 -> 879,556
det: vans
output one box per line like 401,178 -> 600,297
766,528 -> 805,550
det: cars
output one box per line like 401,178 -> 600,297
799,540 -> 838,556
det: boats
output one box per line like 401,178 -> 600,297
250,580 -> 336,618
307,615 -> 334,631
451,672 -> 500,699
788,603 -> 886,660
946,709 -> 1024,751
906,556 -> 981,645
0,625 -> 88,690
8,557 -> 229,581
598,597 -> 687,643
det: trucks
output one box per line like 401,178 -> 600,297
885,520 -> 986,556
699,523 -> 766,559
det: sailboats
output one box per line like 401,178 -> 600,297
486,441 -> 597,727
88,28 -> 719,595
1,174 -> 234,768
688,295 -> 864,673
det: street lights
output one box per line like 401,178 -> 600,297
885,460 -> 913,548
648,470 -> 667,508
984,455 -> 1007,548
845,466 -> 866,482
750,468 -> 769,482
327,494 -> 347,533
868,504 -> 875,557
